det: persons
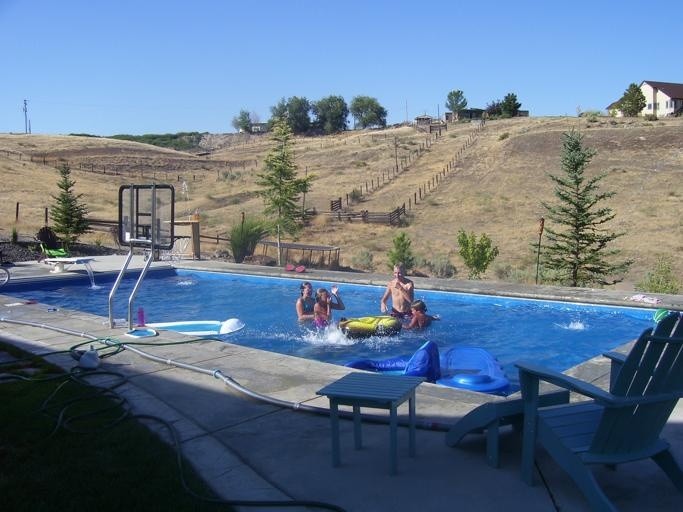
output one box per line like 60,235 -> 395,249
402,300 -> 440,329
313,285 -> 345,335
296,282 -> 315,321
381,263 -> 414,321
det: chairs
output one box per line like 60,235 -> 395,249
513,312 -> 683,511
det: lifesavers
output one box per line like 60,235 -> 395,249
341,316 -> 401,338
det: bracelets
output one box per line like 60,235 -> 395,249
327,303 -> 331,304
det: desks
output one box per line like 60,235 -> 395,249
316,372 -> 428,474
